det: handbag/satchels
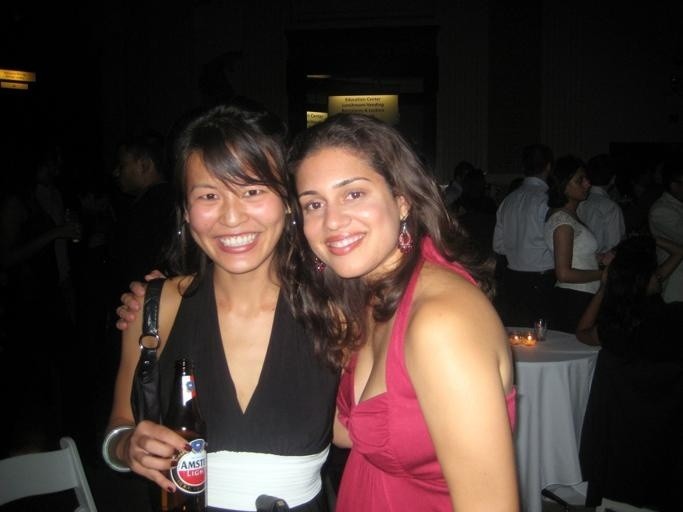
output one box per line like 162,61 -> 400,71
130,279 -> 161,422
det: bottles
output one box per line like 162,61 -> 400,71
162,356 -> 207,511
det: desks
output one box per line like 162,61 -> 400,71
504,327 -> 600,512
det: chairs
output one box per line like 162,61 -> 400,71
0,436 -> 97,511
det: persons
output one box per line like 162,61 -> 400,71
113,110 -> 522,512
78,135 -> 199,305
100,104 -> 342,512
448,131 -> 681,453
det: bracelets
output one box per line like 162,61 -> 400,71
99,425 -> 135,475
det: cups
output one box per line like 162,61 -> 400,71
534,319 -> 547,341
